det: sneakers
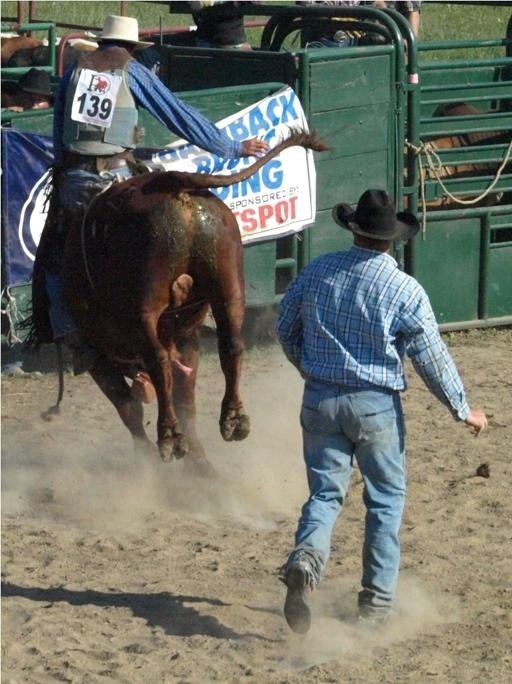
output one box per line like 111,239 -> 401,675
284,563 -> 314,634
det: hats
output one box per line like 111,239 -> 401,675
2,68 -> 56,105
84,15 -> 155,51
332,189 -> 420,241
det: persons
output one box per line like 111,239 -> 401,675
187,1 -> 420,57
37,13 -> 269,377
1,69 -> 57,112
271,188 -> 488,634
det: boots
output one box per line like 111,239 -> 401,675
69,330 -> 101,376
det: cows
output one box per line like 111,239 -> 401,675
62,127 -> 335,478
403,100 -> 512,209
